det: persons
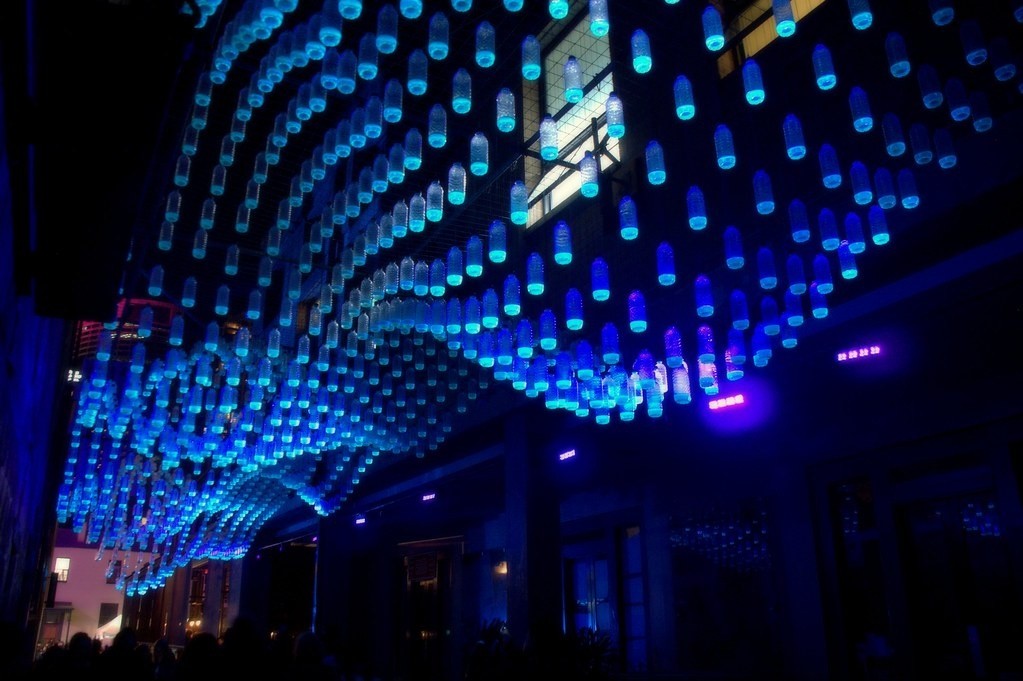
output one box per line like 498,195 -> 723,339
0,615 -> 337,681
